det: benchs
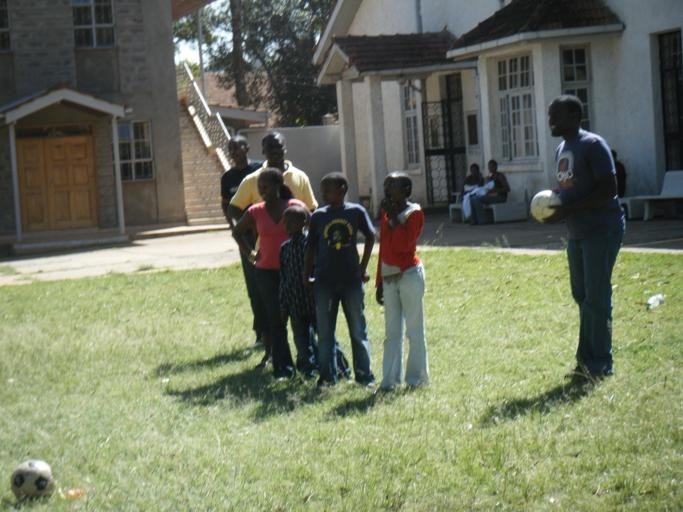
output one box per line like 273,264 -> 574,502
619,197 -> 645,219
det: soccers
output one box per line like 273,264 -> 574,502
10,459 -> 55,504
531,190 -> 561,223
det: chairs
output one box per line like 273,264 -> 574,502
449,172 -> 530,224
631,170 -> 683,221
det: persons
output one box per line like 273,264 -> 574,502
231,168 -> 312,371
461,164 -> 485,223
228,132 -> 322,374
310,172 -> 376,392
543,93 -> 627,376
220,135 -> 266,235
612,150 -> 626,197
273,206 -> 316,383
374,169 -> 429,395
471,160 -> 511,226
